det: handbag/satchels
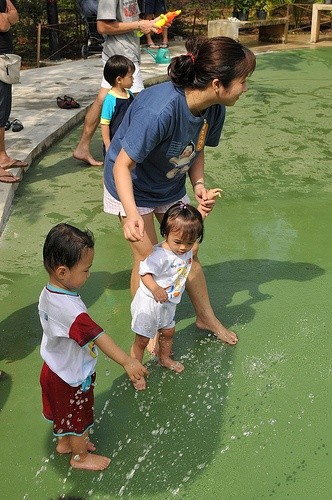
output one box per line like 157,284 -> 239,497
0,53 -> 22,84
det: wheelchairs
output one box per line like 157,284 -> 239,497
81,15 -> 106,60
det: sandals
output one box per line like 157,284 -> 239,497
56,96 -> 79,109
4,119 -> 24,132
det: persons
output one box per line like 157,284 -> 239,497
140,0 -> 170,48
130,187 -> 223,390
103,36 -> 256,356
73,0 -> 172,166
38,223 -> 150,470
100,55 -> 136,159
0,0 -> 29,183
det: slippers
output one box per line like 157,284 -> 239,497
5,159 -> 28,168
145,43 -> 169,49
0,172 -> 24,183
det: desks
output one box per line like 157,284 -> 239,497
311,3 -> 332,42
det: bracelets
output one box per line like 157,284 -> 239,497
192,182 -> 204,191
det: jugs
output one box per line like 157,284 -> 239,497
142,47 -> 171,63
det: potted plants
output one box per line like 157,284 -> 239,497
256,0 -> 272,20
232,0 -> 254,21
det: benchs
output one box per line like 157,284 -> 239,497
208,18 -> 288,44
